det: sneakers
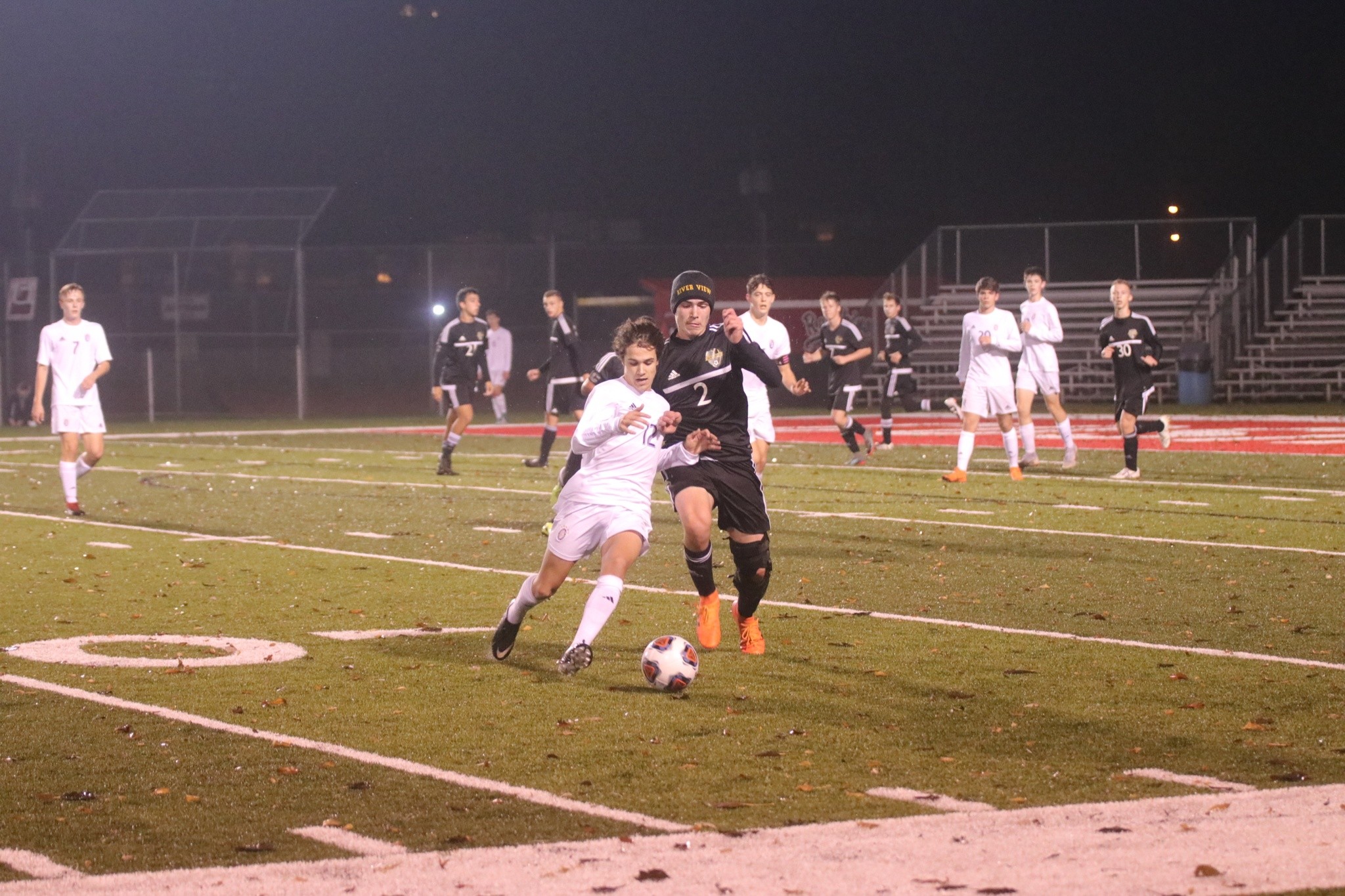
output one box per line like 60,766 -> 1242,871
437,464 -> 459,475
1109,467 -> 1140,480
522,458 -> 548,468
844,458 -> 867,466
696,595 -> 721,649
1009,467 -> 1024,482
942,467 -> 966,482
1156,415 -> 1172,448
64,501 -> 85,516
944,397 -> 962,421
876,442 -> 894,450
436,451 -> 451,468
557,643 -> 593,676
1018,453 -> 1039,467
551,483 -> 563,499
1062,444 -> 1077,470
491,598 -> 523,661
542,521 -> 553,536
732,599 -> 765,655
864,429 -> 875,455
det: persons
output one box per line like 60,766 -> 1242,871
942,276 -> 1023,482
876,293 -> 965,450
802,292 -> 872,467
489,270 -> 809,677
1015,265 -> 1080,470
429,287 -> 489,478
30,283 -> 114,515
486,310 -> 513,425
1098,279 -> 1172,479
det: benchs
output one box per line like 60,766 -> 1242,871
856,274 -> 1345,405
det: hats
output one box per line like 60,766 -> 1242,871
670,271 -> 715,315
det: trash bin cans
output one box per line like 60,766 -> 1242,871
1176,357 -> 1212,406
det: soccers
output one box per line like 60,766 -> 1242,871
641,635 -> 699,691
